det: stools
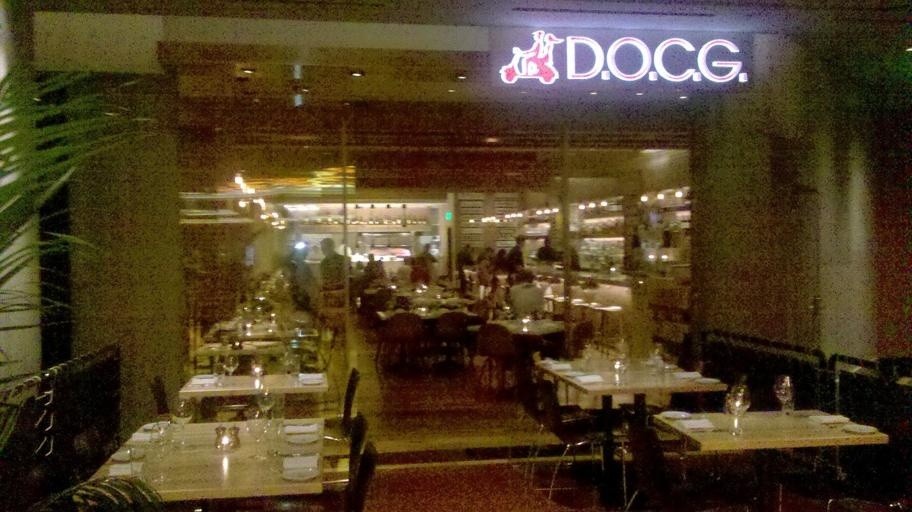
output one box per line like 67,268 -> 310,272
590,302 -> 623,333
572,298 -> 597,320
545,294 -> 564,313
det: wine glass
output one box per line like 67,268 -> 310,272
170,396 -> 192,448
728,383 -> 751,440
246,407 -> 272,461
256,382 -> 273,430
775,374 -> 795,414
150,420 -> 174,480
222,352 -> 240,382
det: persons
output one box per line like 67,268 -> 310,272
318,237 -> 354,291
418,254 -> 440,280
356,261 -> 365,273
506,235 -> 528,271
658,232 -> 673,251
396,256 -> 412,281
422,243 -> 440,265
570,246 -> 580,271
477,246 -> 495,262
411,260 -> 430,282
536,237 -> 560,263
290,240 -> 320,310
455,242 -> 478,278
365,254 -> 386,278
509,268 -> 545,317
495,248 -> 507,269
629,234 -> 648,269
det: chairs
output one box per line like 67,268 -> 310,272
347,410 -> 368,486
510,359 -> 549,480
474,322 -> 514,393
434,311 -> 469,371
323,440 -> 378,512
152,376 -> 170,414
538,379 -> 601,500
324,368 -> 360,428
378,312 -> 425,371
570,321 -> 594,355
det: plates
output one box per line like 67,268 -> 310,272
564,370 -> 585,377
843,424 -> 877,434
696,377 -> 721,384
287,434 -> 318,444
143,423 -> 157,431
677,418 -> 717,431
662,410 -> 691,420
675,371 -> 702,380
551,362 -> 572,370
194,374 -> 213,379
574,375 -> 604,385
284,424 -> 317,433
302,381 -> 322,385
282,454 -> 318,468
809,414 -> 849,424
282,467 -> 319,480
110,461 -> 144,478
111,446 -> 145,461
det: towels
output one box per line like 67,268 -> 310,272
673,371 -> 701,379
193,374 -> 216,380
298,372 -> 325,382
695,378 -> 720,386
281,454 -> 318,470
109,447 -> 146,464
551,363 -> 570,370
678,418 -> 716,433
131,431 -> 162,442
576,374 -> 605,384
564,371 -> 586,377
282,423 -> 318,436
843,421 -> 879,435
807,414 -> 849,426
